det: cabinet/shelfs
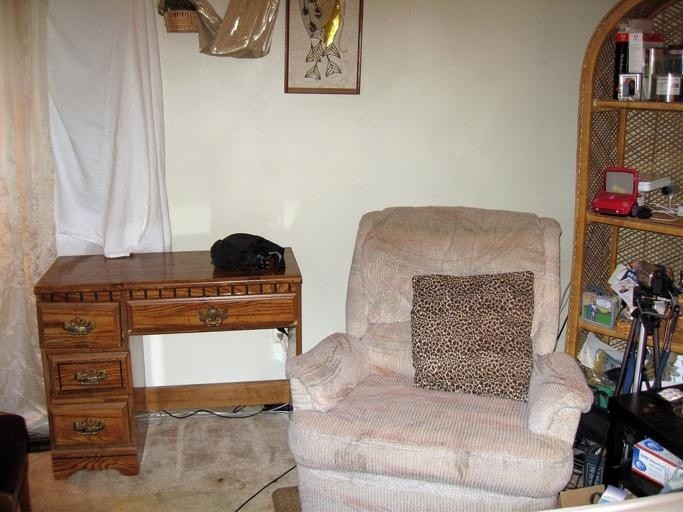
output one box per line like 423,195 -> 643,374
566,2 -> 682,376
32,246 -> 304,477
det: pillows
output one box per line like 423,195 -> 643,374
411,269 -> 535,402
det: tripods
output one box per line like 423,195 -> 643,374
589,297 -> 680,488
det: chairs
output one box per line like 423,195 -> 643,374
279,204 -> 595,510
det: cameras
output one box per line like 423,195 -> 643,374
635,260 -> 665,296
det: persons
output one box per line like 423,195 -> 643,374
627,81 -> 636,96
622,78 -> 631,96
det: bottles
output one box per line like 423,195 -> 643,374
613,33 -> 629,99
648,48 -> 664,102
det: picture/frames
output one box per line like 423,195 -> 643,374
284,1 -> 364,94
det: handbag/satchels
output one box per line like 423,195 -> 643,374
210,233 -> 286,271
